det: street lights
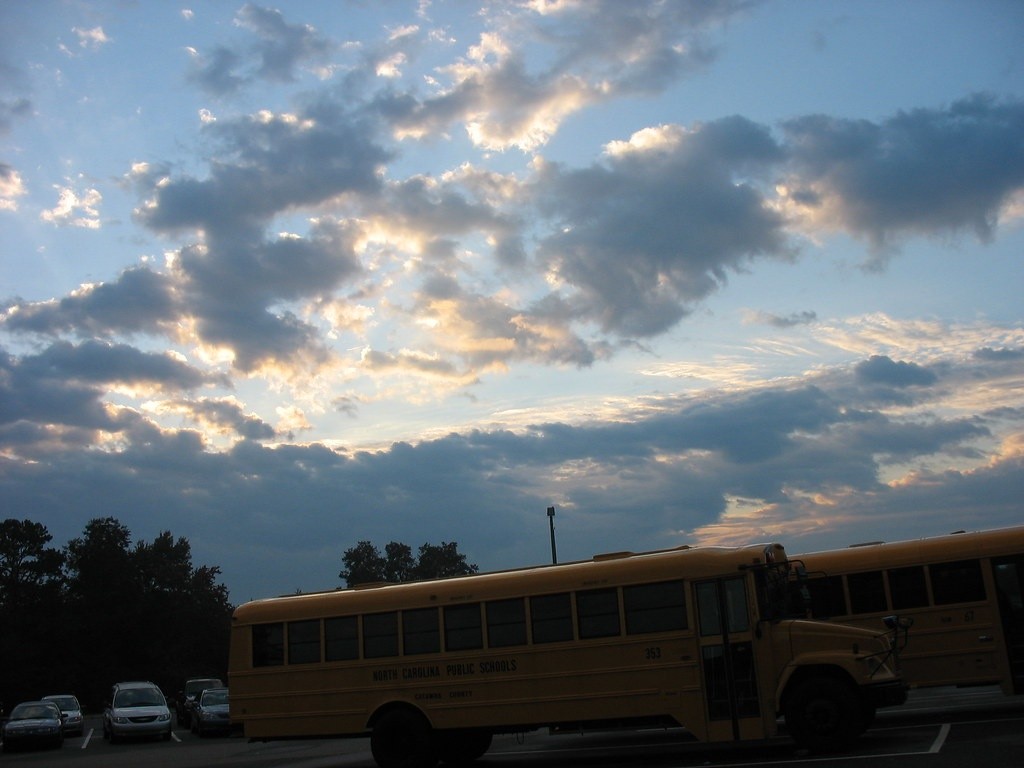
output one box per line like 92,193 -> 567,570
546,506 -> 558,565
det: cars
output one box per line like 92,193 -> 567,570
41,694 -> 84,738
175,679 -> 224,728
0,701 -> 68,751
192,688 -> 243,738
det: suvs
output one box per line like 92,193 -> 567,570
101,681 -> 172,742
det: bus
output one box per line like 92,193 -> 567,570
782,523 -> 1024,713
225,538 -> 912,768
